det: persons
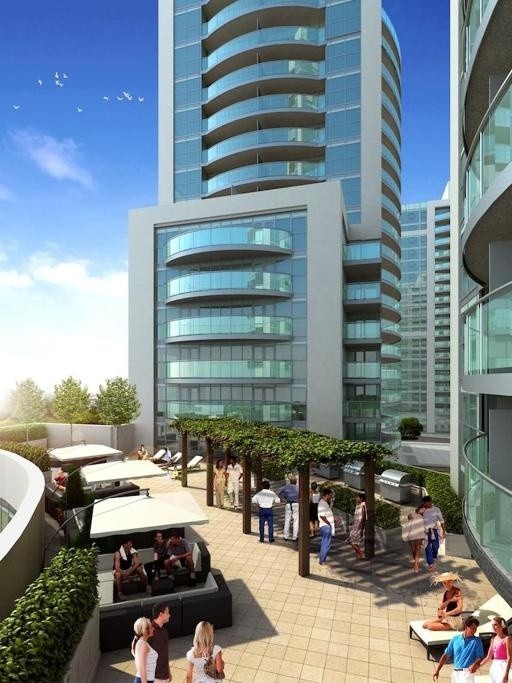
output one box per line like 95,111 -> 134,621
186,621 -> 225,683
153,532 -> 169,581
470,617 -> 512,683
213,457 -> 369,564
112,539 -> 152,600
164,532 -> 196,580
423,572 -> 465,631
130,617 -> 159,683
138,445 -> 171,463
147,602 -> 172,683
433,616 -> 484,683
408,506 -> 426,574
408,496 -> 447,568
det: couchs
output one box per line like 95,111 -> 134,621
94,568 -> 232,651
82,482 -> 139,506
92,542 -> 211,594
44,481 -> 64,522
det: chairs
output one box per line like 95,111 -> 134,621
408,591 -> 511,661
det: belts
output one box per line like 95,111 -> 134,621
455,669 -> 463,671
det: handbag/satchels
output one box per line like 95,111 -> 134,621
204,658 -> 225,679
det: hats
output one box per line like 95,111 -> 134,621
431,572 -> 460,582
